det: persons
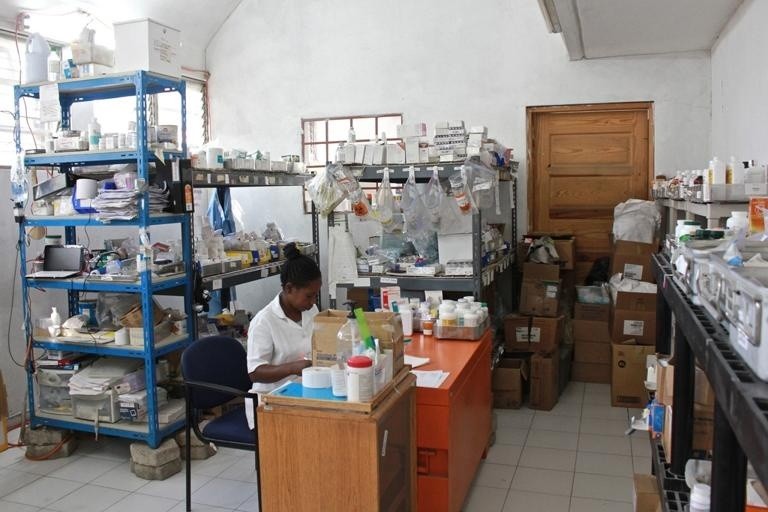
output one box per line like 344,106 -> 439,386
244,240 -> 321,434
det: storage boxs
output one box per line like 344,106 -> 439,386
34,366 -> 122,426
110,17 -> 182,80
656,354 -> 714,463
491,232 -> 660,411
631,472 -> 659,512
311,309 -> 404,377
665,233 -> 766,381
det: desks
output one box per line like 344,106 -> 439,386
403,327 -> 493,512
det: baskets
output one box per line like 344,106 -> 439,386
118,300 -> 163,327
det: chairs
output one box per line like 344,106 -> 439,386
180,334 -> 262,512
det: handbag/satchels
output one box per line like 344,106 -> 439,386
526,236 -> 559,264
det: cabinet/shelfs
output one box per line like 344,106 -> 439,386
328,161 -> 519,311
12,70 -> 199,450
657,197 -> 751,239
191,167 -> 321,311
651,251 -> 768,511
255,362 -> 420,512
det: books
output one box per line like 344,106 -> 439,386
35,349 -> 187,425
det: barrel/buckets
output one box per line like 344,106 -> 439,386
20,32 -> 51,86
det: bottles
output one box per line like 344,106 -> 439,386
48,47 -> 61,80
347,126 -> 357,144
418,303 -> 434,336
437,294 -> 488,328
674,219 -> 700,242
344,356 -> 374,404
674,169 -> 707,201
89,116 -> 126,153
336,142 -> 346,163
45,133 -> 56,154
50,306 -> 61,326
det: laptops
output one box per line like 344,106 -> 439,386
25,245 -> 84,279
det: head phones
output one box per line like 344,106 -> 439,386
90,252 -> 122,271
